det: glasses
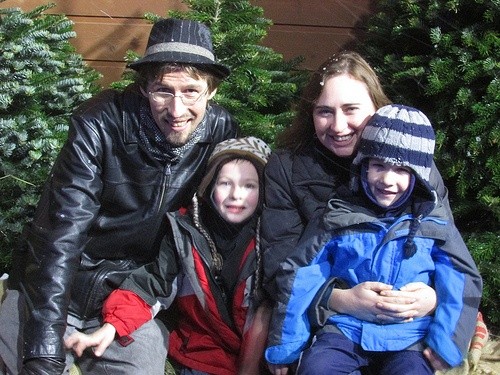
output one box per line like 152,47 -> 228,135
144,83 -> 213,107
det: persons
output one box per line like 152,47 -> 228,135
0,18 -> 238,375
267,103 -> 483,375
259,50 -> 453,375
66,136 -> 271,375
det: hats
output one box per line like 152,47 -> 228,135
126,17 -> 232,80
190,136 -> 273,303
349,104 -> 438,260
